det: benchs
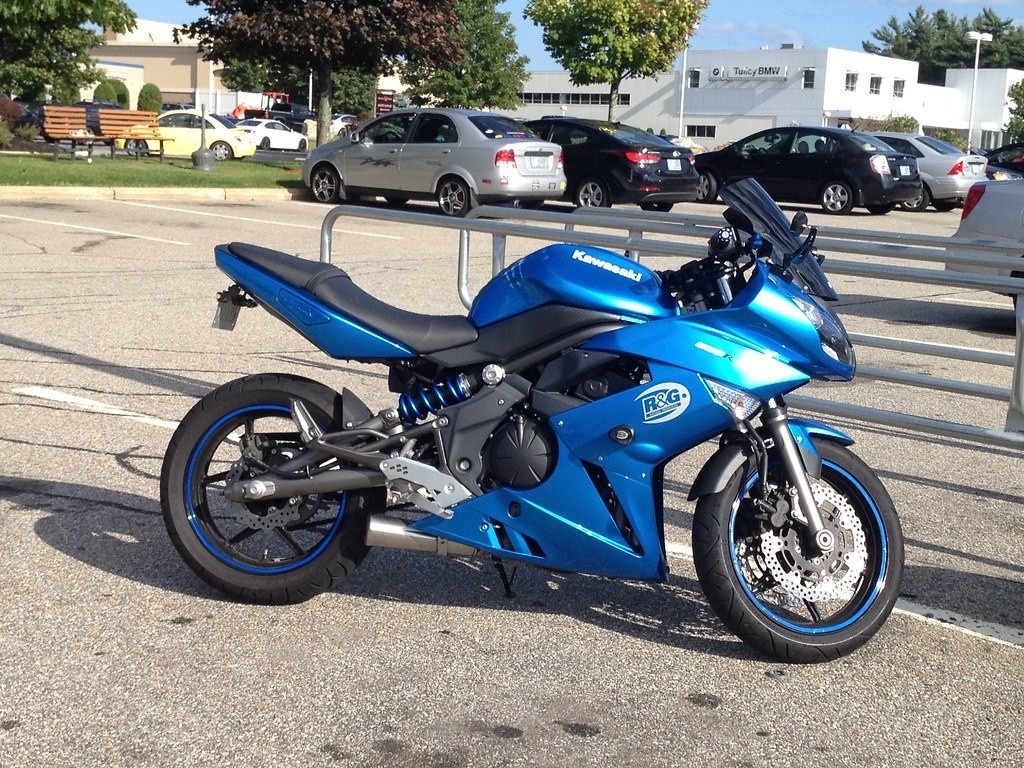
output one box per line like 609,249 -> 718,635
40,104 -> 110,165
96,109 -> 175,164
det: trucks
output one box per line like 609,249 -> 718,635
243,103 -> 315,133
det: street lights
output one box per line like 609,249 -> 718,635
677,20 -> 699,137
964,30 -> 993,157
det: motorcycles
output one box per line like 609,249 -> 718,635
158,179 -> 907,666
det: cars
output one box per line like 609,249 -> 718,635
114,109 -> 256,159
963,142 -> 1024,182
694,126 -> 923,215
68,100 -> 122,147
519,116 -> 702,213
219,114 -> 239,126
325,112 -> 358,134
302,108 -> 567,216
9,95 -> 65,143
864,130 -> 988,214
160,103 -> 195,116
934,179 -> 1023,296
235,117 -> 308,151
